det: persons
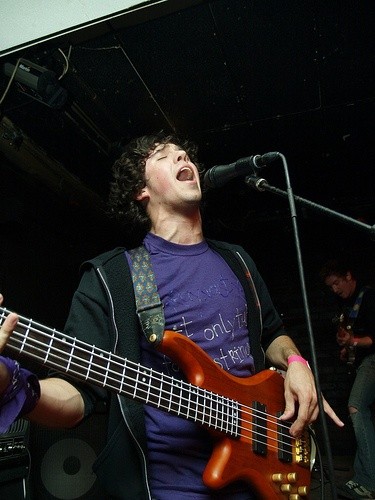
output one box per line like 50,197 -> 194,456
0,108 -> 348,500
313,255 -> 375,500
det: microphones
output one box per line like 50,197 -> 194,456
203,152 -> 280,188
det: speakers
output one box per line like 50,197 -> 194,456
28,401 -> 105,500
0,465 -> 37,500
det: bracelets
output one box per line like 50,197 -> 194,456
286,352 -> 310,370
352,337 -> 360,346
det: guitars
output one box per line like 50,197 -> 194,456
0,304 -> 313,500
337,313 -> 348,361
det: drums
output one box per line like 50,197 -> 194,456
39,436 -> 98,500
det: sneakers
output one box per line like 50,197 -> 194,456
337,485 -> 375,500
336,480 -> 359,492
